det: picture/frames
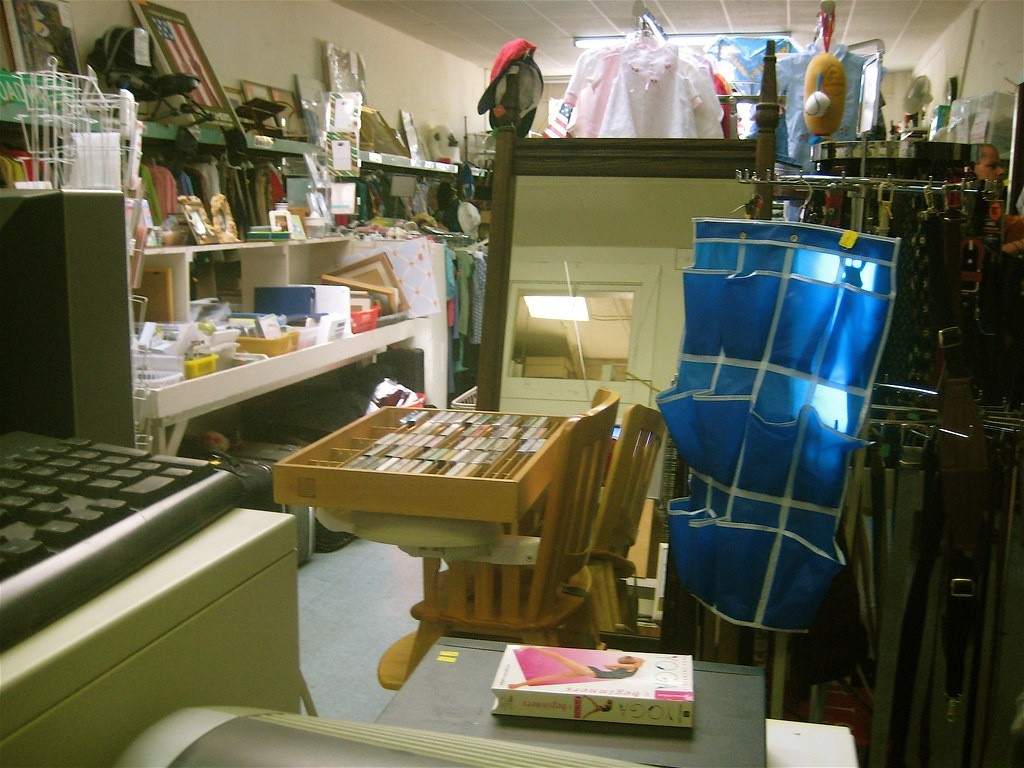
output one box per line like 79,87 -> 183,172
129,0 -> 433,162
317,253 -> 412,326
125,193 -> 308,246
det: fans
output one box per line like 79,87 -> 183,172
903,74 -> 935,131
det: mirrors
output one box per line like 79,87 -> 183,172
437,38 -> 783,660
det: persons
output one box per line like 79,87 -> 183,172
508,647 -> 647,689
583,696 -> 613,719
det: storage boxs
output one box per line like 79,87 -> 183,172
136,303 -> 408,380
490,644 -> 694,729
929,90 -> 1016,161
273,404 -> 579,523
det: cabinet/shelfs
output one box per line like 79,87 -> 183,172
60,186 -> 450,458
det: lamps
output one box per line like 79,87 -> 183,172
572,30 -> 795,48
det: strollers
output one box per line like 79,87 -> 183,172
86,25 -> 216,127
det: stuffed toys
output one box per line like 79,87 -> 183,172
801,5 -> 848,146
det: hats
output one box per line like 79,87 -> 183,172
457,200 -> 482,241
488,55 -> 545,137
477,39 -> 538,116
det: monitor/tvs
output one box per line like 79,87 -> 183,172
0,189 -> 136,450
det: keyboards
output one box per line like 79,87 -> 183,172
0,431 -> 246,657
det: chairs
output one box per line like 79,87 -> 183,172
313,389 -> 620,696
588,400 -> 669,636
